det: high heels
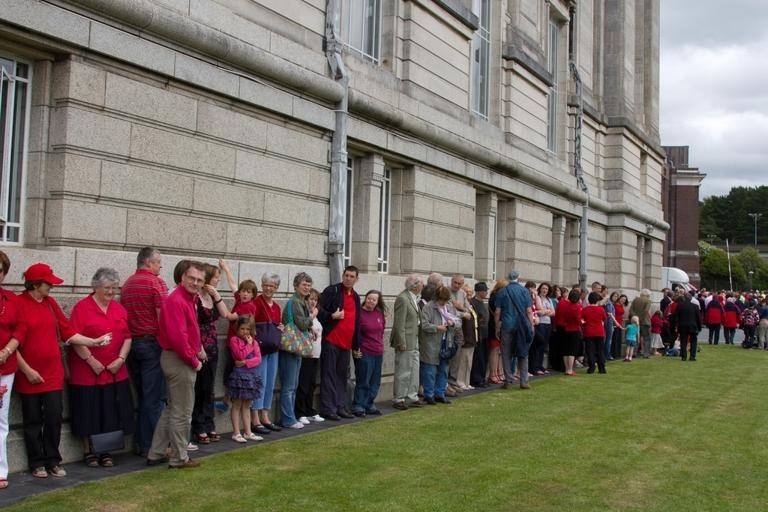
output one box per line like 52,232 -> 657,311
488,376 -> 503,384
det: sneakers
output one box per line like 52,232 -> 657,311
460,386 -> 469,390
307,415 -> 324,422
288,422 -> 304,429
411,400 -> 427,406
624,356 -> 629,360
467,385 -> 475,390
447,386 -> 456,393
33,466 -> 47,478
475,383 -> 489,389
629,357 -> 632,361
451,385 -> 462,393
392,401 -> 408,409
186,443 -> 199,451
299,417 -> 310,425
48,465 -> 66,477
500,383 -> 512,390
0,477 -> 8,488
519,384 -> 530,388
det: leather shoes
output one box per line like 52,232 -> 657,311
535,371 -> 544,375
682,357 -> 686,361
325,414 -> 341,420
353,411 -> 366,417
370,408 -> 382,415
423,398 -> 437,405
434,397 -> 451,404
338,409 -> 354,418
690,356 -> 696,361
147,454 -> 170,465
168,458 -> 201,469
544,370 -> 551,374
251,421 -> 271,433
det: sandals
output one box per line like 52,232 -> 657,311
210,431 -> 221,441
198,433 -> 210,443
83,453 -> 99,467
232,434 -> 247,442
100,453 -> 114,466
261,420 -> 282,431
243,433 -> 264,441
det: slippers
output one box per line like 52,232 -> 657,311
567,370 -> 576,376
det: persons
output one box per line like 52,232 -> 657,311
582,292 -> 608,374
524,280 -> 545,326
0,250 -> 28,490
278,272 -> 318,430
418,272 -> 444,308
620,316 -> 640,361
662,287 -> 684,304
294,288 -> 325,425
553,289 -> 583,376
688,288 -> 768,311
553,281 -> 607,298
228,316 -> 264,443
740,301 -> 759,348
533,283 -> 555,375
630,289 -> 651,358
722,297 -> 740,345
605,291 -> 620,360
389,276 -> 427,410
455,283 -> 481,391
470,282 -> 491,388
15,262 -> 112,478
447,274 -> 471,396
676,293 -> 702,361
612,295 -> 629,356
757,299 -> 768,350
191,264 -> 227,443
120,247 -> 168,458
146,263 -> 207,469
353,290 -> 387,416
498,348 -> 506,381
495,271 -> 533,389
420,286 -> 462,405
68,267 -> 133,466
218,257 -> 258,387
316,265 -> 361,420
251,272 -> 282,434
173,259 -> 208,451
488,278 -> 509,384
650,310 -> 665,355
705,295 -> 723,345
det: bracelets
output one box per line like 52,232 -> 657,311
118,355 -> 126,364
84,354 -> 93,362
5,346 -> 12,355
214,298 -> 223,304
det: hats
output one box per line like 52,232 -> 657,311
25,263 -> 64,285
508,271 -> 520,280
474,282 -> 489,291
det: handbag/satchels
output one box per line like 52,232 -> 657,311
440,324 -> 458,359
506,287 -> 533,345
254,322 -> 281,357
280,298 -> 313,357
88,368 -> 125,454
